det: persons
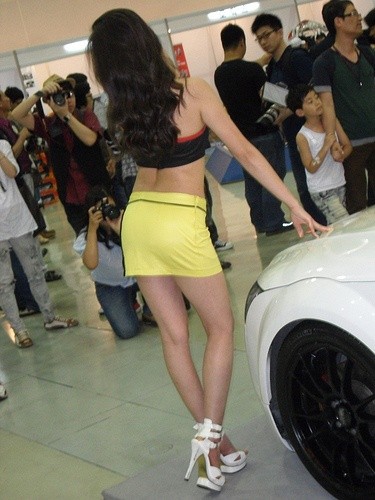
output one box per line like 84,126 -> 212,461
214,22 -> 295,236
85,8 -> 335,492
0,71 -> 235,348
284,83 -> 354,227
251,0 -> 375,226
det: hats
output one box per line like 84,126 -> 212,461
67,73 -> 87,83
42,74 -> 76,102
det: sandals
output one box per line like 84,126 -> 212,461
15,329 -> 33,348
44,316 -> 78,331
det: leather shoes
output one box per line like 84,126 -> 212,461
266,221 -> 295,237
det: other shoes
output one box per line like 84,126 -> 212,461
220,262 -> 231,269
0,385 -> 8,400
98,305 -> 104,315
19,308 -> 41,317
42,248 -> 48,257
39,235 -> 49,244
142,316 -> 158,327
133,302 -> 141,313
45,271 -> 63,283
213,240 -> 232,251
42,230 -> 56,238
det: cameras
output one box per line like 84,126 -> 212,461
45,86 -> 73,106
92,198 -> 121,220
255,84 -> 289,132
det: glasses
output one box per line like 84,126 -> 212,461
344,11 -> 361,18
255,28 -> 278,42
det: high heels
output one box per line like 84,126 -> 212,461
184,417 -> 226,492
193,423 -> 248,474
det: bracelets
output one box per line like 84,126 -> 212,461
63,113 -> 72,124
21,132 -> 26,139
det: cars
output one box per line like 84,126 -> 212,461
242,202 -> 375,500
